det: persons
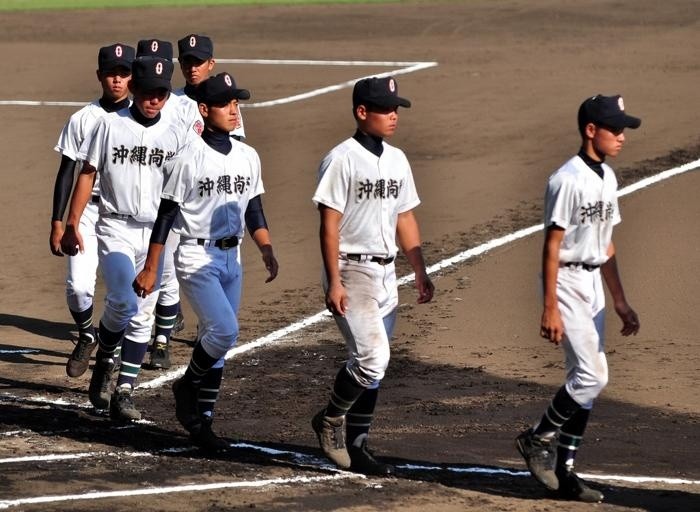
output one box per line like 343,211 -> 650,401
513,92 -> 641,504
129,70 -> 280,448
310,73 -> 434,475
61,54 -> 201,427
168,28 -> 249,347
48,44 -> 134,380
129,38 -> 188,371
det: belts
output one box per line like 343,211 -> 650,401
563,261 -> 602,272
89,196 -> 101,203
341,253 -> 396,266
180,236 -> 241,248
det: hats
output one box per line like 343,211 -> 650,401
352,76 -> 412,113
195,71 -> 251,107
176,34 -> 214,62
130,55 -> 175,96
135,39 -> 173,63
96,43 -> 136,74
577,94 -> 642,132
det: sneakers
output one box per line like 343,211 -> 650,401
310,408 -> 355,469
551,465 -> 607,503
65,330 -> 99,378
172,378 -> 204,435
193,417 -> 232,451
172,313 -> 186,333
513,428 -> 562,492
344,441 -> 397,476
108,387 -> 142,422
150,336 -> 172,370
87,349 -> 116,410
